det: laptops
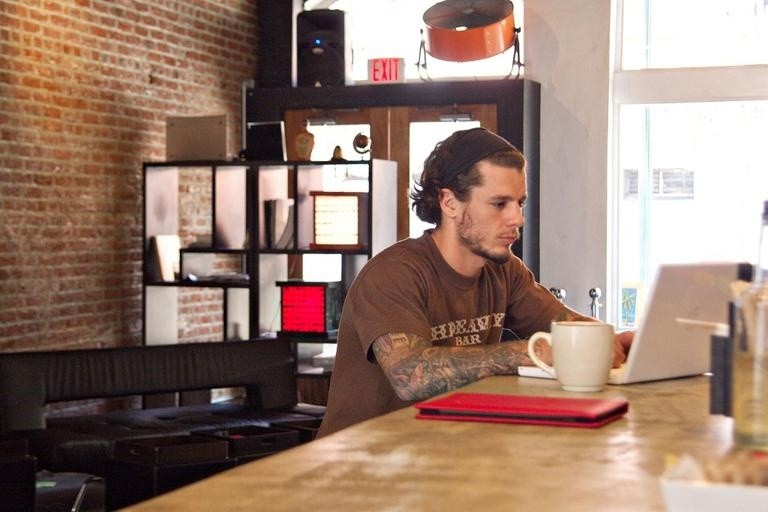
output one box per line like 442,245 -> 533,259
518,262 -> 740,386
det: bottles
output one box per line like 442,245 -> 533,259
729,200 -> 768,452
295,120 -> 314,162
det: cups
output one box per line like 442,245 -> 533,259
528,320 -> 614,392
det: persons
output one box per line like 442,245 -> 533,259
316,125 -> 638,443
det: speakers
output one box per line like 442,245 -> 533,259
297,9 -> 345,86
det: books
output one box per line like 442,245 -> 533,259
413,391 -> 629,428
267,196 -> 295,251
148,234 -> 181,281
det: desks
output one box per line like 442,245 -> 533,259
122,372 -> 768,510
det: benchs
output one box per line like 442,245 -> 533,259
0,338 -> 327,478
